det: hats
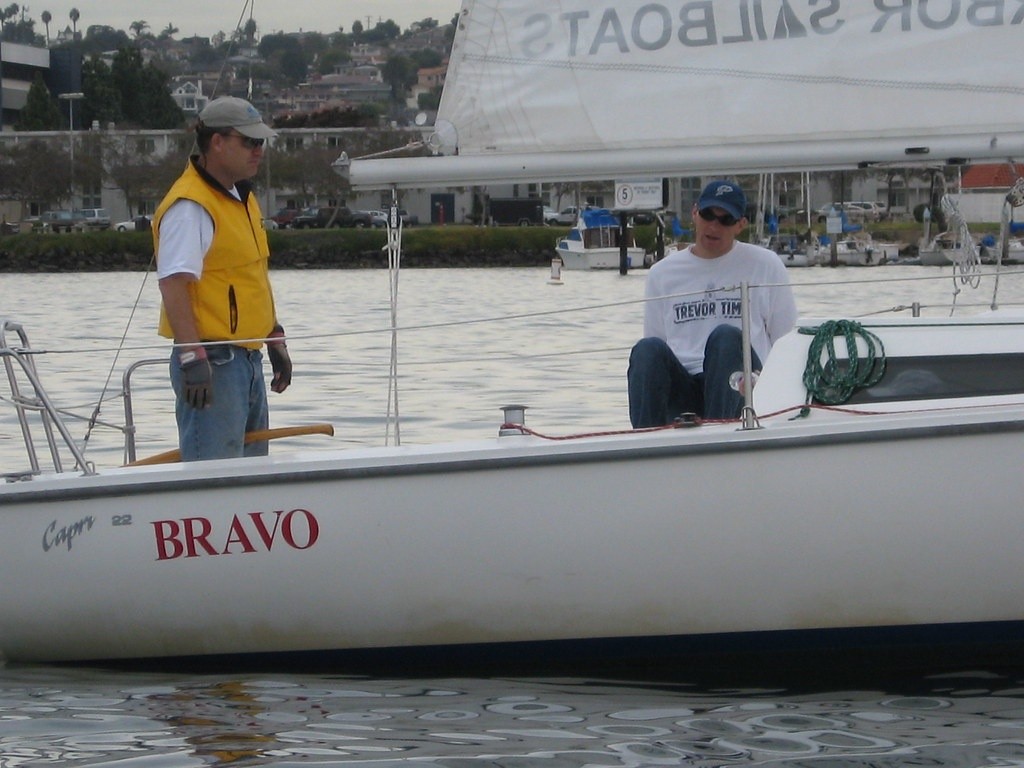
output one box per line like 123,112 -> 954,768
199,95 -> 279,139
695,179 -> 745,220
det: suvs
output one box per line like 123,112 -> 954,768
72,207 -> 111,230
35,208 -> 78,232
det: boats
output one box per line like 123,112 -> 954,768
554,208 -> 646,272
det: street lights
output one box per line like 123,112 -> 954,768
58,92 -> 85,202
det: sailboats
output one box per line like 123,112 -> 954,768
739,170 -> 1024,269
0,0 -> 1024,691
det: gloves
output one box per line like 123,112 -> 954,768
263,325 -> 292,393
177,347 -> 213,411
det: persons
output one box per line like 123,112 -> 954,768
626,181 -> 797,429
151,96 -> 292,462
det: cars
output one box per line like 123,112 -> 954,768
267,205 -> 420,229
112,214 -> 154,231
544,205 -> 582,227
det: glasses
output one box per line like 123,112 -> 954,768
215,131 -> 265,149
699,207 -> 736,226
